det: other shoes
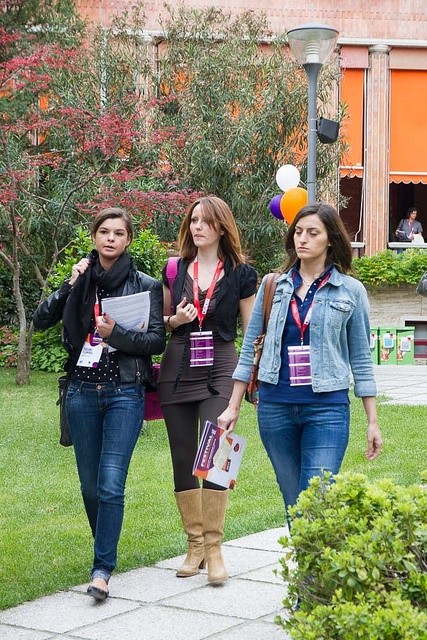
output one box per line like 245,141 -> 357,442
87,578 -> 109,601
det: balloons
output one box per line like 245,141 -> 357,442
276,164 -> 300,192
280,187 -> 309,224
269,195 -> 285,220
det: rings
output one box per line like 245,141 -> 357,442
188,309 -> 193,313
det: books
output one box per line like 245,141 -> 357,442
191,420 -> 247,491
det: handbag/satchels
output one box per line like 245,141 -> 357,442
244,273 -> 281,405
58,376 -> 74,446
141,363 -> 165,421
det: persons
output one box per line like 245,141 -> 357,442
216,203 -> 382,609
157,197 -> 257,582
32,208 -> 166,599
395,207 -> 423,254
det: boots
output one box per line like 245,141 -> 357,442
173,489 -> 206,577
201,488 -> 228,582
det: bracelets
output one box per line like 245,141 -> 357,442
167,316 -> 173,328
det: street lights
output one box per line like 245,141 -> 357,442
286,25 -> 338,205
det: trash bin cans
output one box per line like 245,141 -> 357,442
369,326 -> 416,365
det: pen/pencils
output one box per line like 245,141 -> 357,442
103,312 -> 106,323
